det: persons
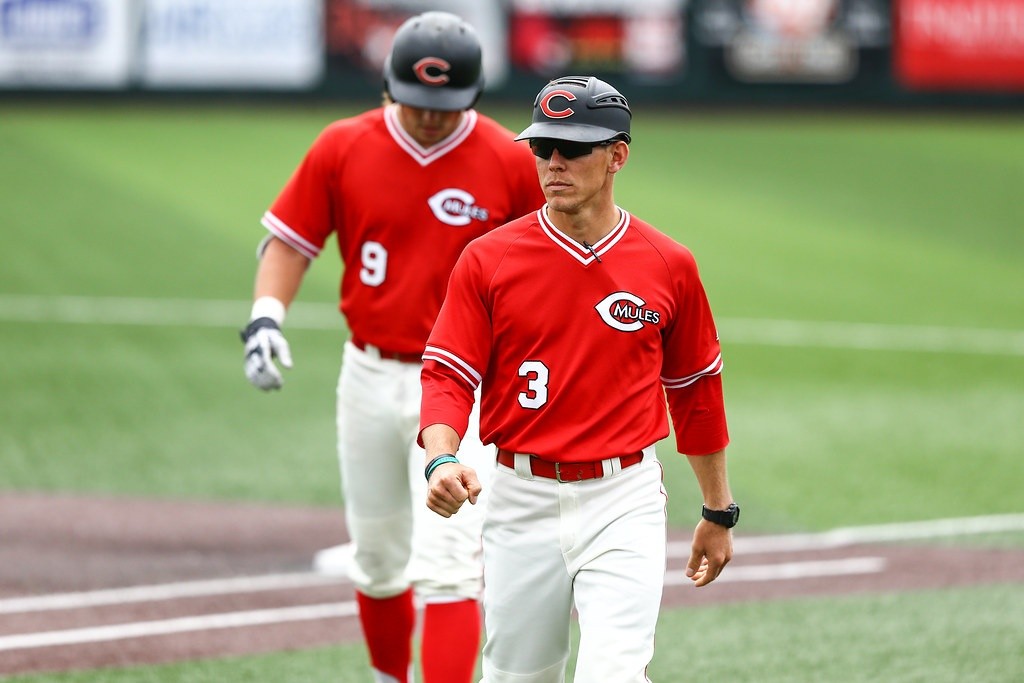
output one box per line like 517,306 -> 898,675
415,75 -> 741,683
239,9 -> 548,683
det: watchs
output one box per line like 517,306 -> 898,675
702,501 -> 740,530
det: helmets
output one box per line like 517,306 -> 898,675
383,10 -> 485,112
513,75 -> 632,145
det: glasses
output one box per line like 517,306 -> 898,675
529,137 -> 623,160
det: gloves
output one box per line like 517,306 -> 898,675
239,317 -> 294,391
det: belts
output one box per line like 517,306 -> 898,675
497,449 -> 644,484
352,334 -> 422,363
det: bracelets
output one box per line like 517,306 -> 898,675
239,317 -> 279,344
424,453 -> 460,483
